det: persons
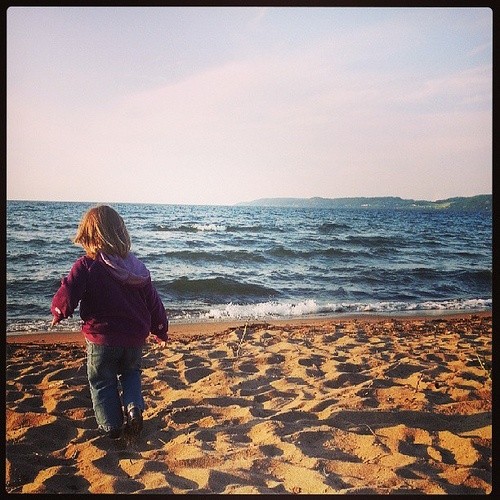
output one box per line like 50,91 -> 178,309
49,205 -> 170,440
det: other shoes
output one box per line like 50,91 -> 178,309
97,424 -> 122,441
124,407 -> 144,437
